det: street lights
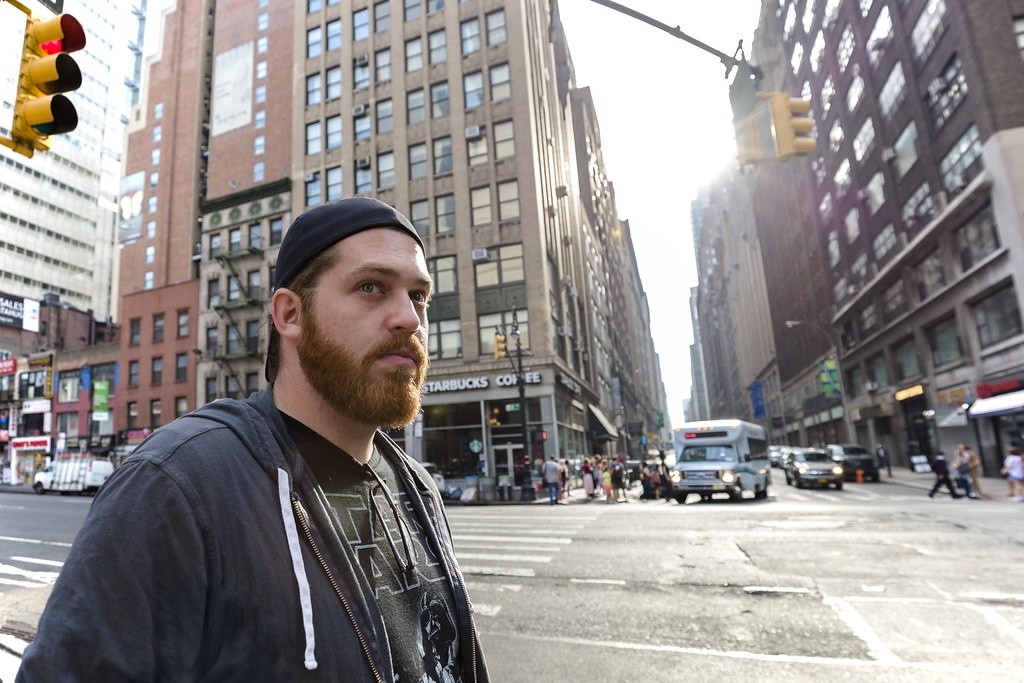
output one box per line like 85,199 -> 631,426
509,304 -> 536,500
786,320 -> 855,445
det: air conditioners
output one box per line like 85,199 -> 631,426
881,147 -> 894,162
464,126 -> 481,141
356,156 -> 370,170
568,287 -> 578,297
193,349 -> 204,361
558,325 -> 572,337
355,54 -> 368,68
351,104 -> 365,118
471,248 -> 490,262
928,78 -> 946,95
948,175 -> 966,194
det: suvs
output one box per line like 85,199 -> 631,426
827,443 -> 882,481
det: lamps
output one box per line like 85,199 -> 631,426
78,336 -> 86,345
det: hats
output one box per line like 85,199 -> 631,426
265,198 -> 425,384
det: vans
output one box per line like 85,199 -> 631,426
32,457 -> 115,495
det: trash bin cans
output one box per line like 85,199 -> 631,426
498,476 -> 513,501
478,477 -> 498,500
465,475 -> 479,489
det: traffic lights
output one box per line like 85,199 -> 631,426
534,431 -> 546,444
736,114 -> 761,169
12,10 -> 85,153
495,334 -> 506,362
771,92 -> 817,160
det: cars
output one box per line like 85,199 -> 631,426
419,463 -> 450,495
784,450 -> 845,488
767,445 -> 821,464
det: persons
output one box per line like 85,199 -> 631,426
955,443 -> 1024,499
928,451 -> 964,500
638,452 -> 674,504
14,197 -> 490,683
876,443 -> 887,472
540,454 -> 630,504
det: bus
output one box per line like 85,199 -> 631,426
666,418 -> 770,503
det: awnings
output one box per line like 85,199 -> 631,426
967,389 -> 1024,416
